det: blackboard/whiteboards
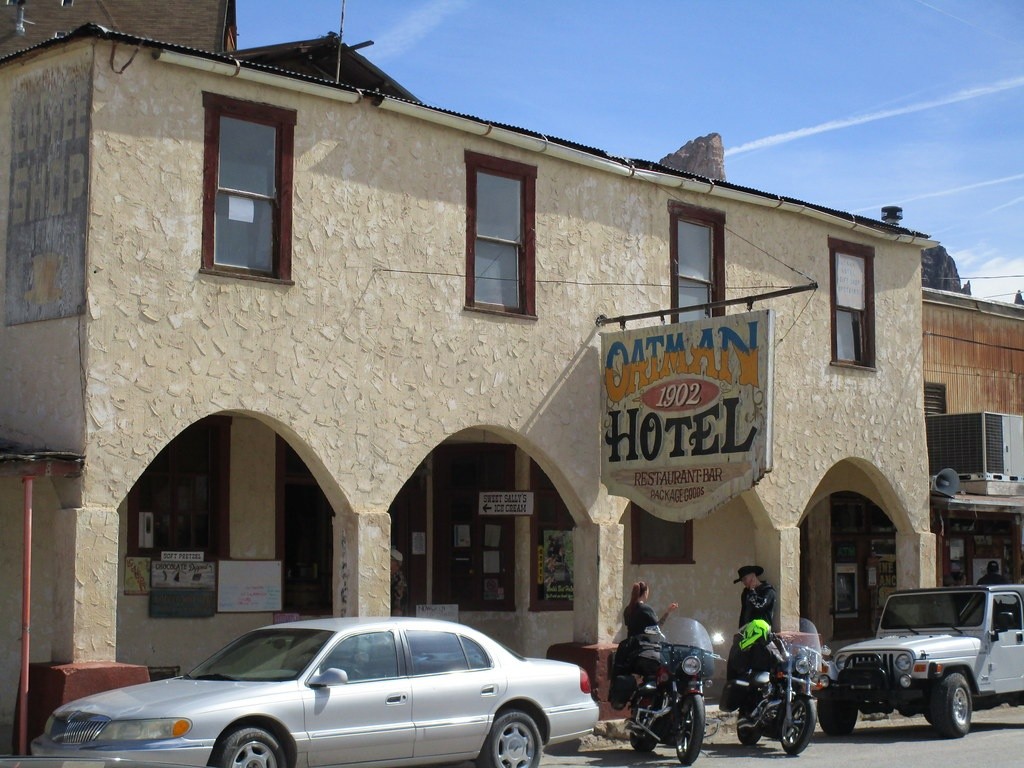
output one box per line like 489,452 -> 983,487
214,557 -> 285,614
415,603 -> 459,623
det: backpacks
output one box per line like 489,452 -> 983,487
614,632 -> 651,674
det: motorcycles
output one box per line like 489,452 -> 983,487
607,617 -> 728,766
718,618 -> 831,756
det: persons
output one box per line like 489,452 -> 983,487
390,547 -> 411,618
976,561 -> 1008,586
623,581 -> 678,638
734,566 -> 776,633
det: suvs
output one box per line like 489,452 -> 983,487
817,582 -> 1023,740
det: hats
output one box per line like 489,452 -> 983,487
733,566 -> 765,584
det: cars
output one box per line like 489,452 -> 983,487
31,616 -> 601,768
